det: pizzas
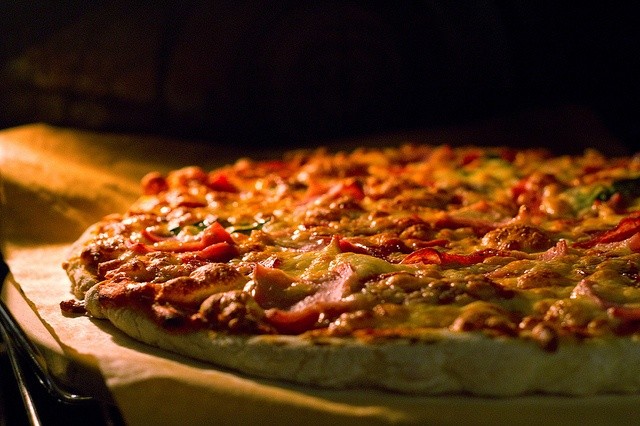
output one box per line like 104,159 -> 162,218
59,142 -> 640,398
